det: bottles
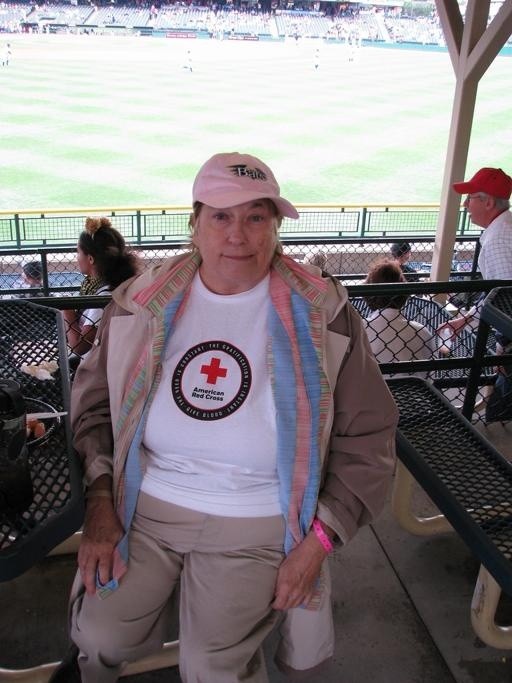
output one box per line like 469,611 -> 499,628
0,378 -> 34,515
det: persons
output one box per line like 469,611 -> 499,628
354,256 -> 436,385
21,215 -> 141,406
389,241 -> 422,282
10,258 -> 56,297
301,250 -> 328,272
433,166 -> 511,421
65,149 -> 400,682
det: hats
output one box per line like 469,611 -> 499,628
192,152 -> 299,219
452,168 -> 512,200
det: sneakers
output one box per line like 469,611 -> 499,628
47,643 -> 81,683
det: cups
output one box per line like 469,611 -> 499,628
438,327 -> 455,356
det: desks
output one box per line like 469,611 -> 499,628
0,298 -> 86,582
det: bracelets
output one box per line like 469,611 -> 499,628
311,517 -> 336,555
82,487 -> 113,499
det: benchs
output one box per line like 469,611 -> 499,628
382,376 -> 511,650
